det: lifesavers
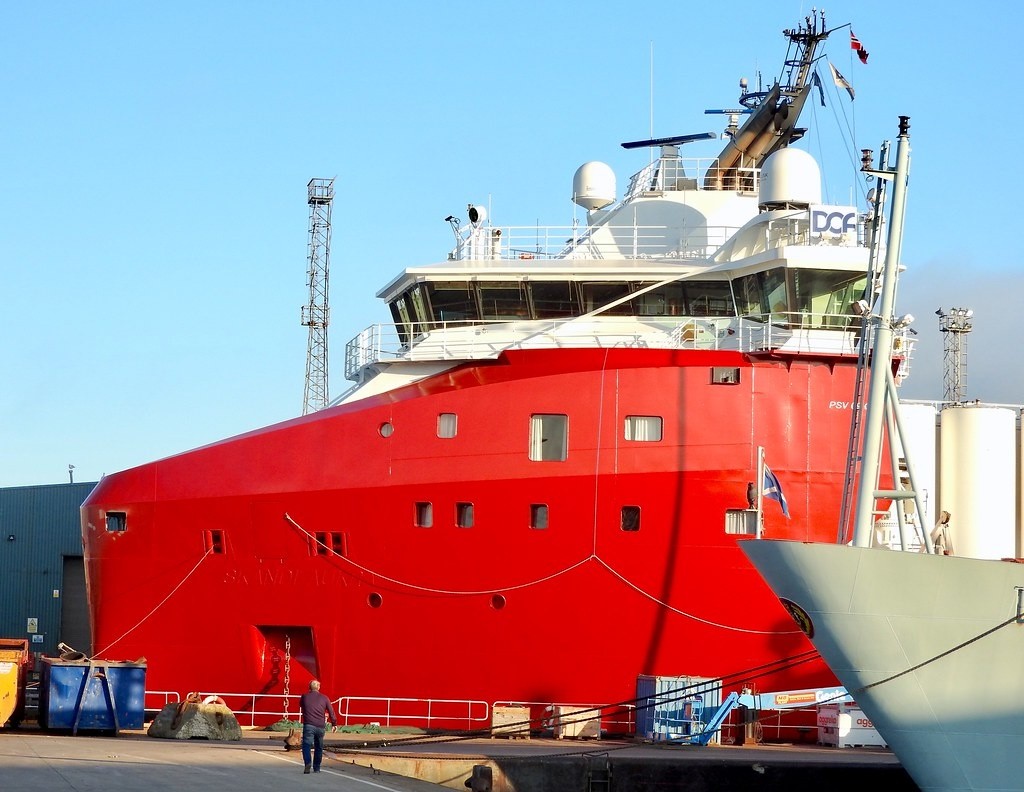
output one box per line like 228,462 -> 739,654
539,705 -> 555,731
202,695 -> 226,704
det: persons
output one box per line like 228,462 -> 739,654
300,681 -> 336,773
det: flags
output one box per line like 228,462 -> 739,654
814,72 -> 826,106
763,467 -> 789,518
850,31 -> 869,65
829,62 -> 855,101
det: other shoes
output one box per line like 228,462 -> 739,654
314,770 -> 320,773
304,764 -> 311,774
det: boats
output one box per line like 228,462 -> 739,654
77,7 -> 914,734
734,112 -> 1024,792
